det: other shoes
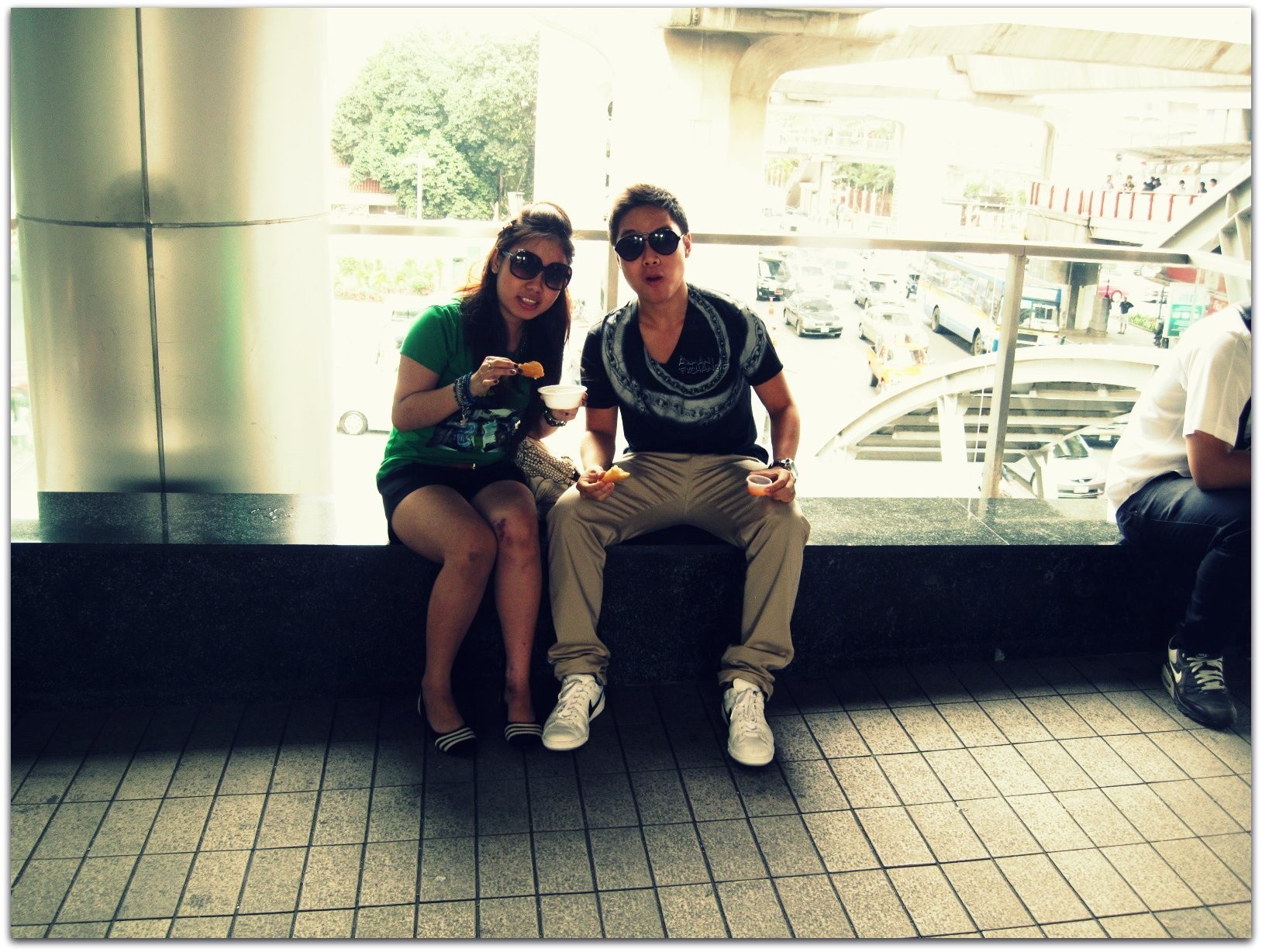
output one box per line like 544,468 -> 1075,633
500,688 -> 542,750
417,691 -> 480,757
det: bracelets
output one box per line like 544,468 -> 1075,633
452,371 -> 476,428
542,406 -> 567,426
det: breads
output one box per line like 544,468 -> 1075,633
516,361 -> 544,379
597,465 -> 630,483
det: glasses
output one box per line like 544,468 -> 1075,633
613,228 -> 683,261
501,249 -> 573,292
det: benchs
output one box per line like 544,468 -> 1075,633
9,496 -> 1125,708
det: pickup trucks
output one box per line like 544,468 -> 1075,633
867,339 -> 927,388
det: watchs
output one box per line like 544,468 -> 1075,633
767,458 -> 799,479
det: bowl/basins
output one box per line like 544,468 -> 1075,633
746,475 -> 773,496
537,384 -> 588,410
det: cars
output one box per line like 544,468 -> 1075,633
784,291 -> 844,339
756,257 -> 796,298
854,278 -> 896,311
855,305 -> 929,357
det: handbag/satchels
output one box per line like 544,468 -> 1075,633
516,435 -> 581,519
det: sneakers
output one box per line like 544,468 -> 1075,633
1162,633 -> 1237,731
541,674 -> 606,750
722,677 -> 775,771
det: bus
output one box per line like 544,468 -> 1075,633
916,252 -> 1069,359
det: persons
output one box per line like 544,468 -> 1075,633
542,186 -> 809,765
1103,175 -> 1218,194
1119,297 -> 1133,335
1104,298 -> 1252,730
374,201 -> 590,758
